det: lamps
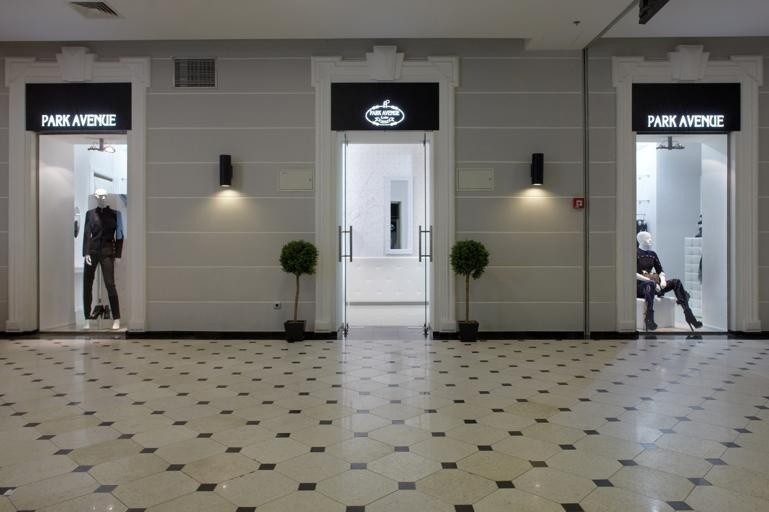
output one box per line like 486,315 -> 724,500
531,151 -> 547,189
218,153 -> 234,187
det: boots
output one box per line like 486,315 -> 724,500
685,308 -> 702,332
645,312 -> 657,332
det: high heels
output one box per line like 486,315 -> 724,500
88,305 -> 110,319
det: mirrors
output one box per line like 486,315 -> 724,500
88,194 -> 127,239
384,176 -> 411,254
74,206 -> 81,238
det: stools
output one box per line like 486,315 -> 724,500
636,296 -> 676,330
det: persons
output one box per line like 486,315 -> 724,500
636,230 -> 703,333
79,187 -> 125,331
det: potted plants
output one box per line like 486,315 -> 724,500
279,239 -> 319,344
449,240 -> 490,341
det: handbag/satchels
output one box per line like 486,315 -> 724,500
643,272 -> 659,285
